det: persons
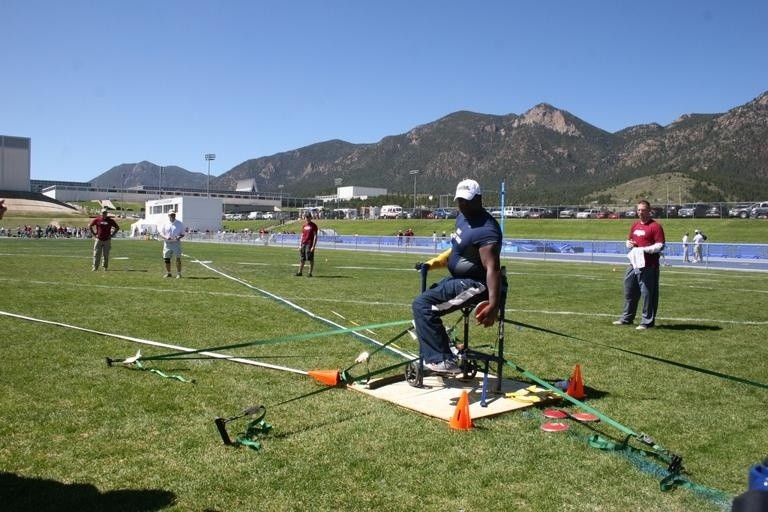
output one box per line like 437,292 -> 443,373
450,232 -> 454,238
0,199 -> 7,219
441,231 -> 446,249
294,213 -> 318,277
432,231 -> 438,242
159,209 -> 186,279
88,209 -> 120,271
612,200 -> 666,330
397,229 -> 413,248
683,229 -> 704,263
412,180 -> 503,373
0,223 -> 92,239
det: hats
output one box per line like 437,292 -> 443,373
100,209 -> 108,213
304,211 -> 312,217
453,178 -> 482,202
168,209 -> 176,215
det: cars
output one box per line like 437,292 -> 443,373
481,205 -> 722,221
400,206 -> 434,220
131,213 -> 142,218
219,208 -> 358,225
726,200 -> 768,219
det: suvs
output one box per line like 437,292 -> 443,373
432,207 -> 458,220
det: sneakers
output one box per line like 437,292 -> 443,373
613,320 -> 623,325
163,272 -> 182,279
636,325 -> 646,330
91,267 -> 108,272
429,358 -> 461,374
307,272 -> 313,277
295,272 -> 303,276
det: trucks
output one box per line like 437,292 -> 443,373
379,204 -> 409,220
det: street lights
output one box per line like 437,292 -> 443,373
278,184 -> 285,210
409,168 -> 422,209
119,173 -> 127,219
158,165 -> 169,199
333,177 -> 345,221
204,153 -> 216,199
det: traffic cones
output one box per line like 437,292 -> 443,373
447,387 -> 474,430
565,362 -> 590,398
305,367 -> 341,387
